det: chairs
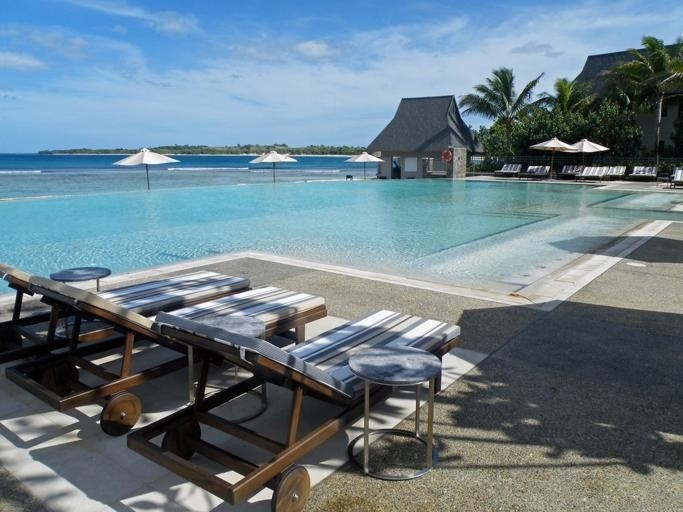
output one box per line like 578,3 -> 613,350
492,162 -> 682,190
0,272 -> 326,438
121,304 -> 462,511
0,256 -> 249,379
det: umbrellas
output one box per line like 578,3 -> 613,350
344,152 -> 385,179
248,151 -> 298,184
113,148 -> 181,190
563,138 -> 611,170
529,136 -> 577,179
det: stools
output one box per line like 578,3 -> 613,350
46,266 -> 109,291
346,344 -> 443,479
193,314 -> 267,424
